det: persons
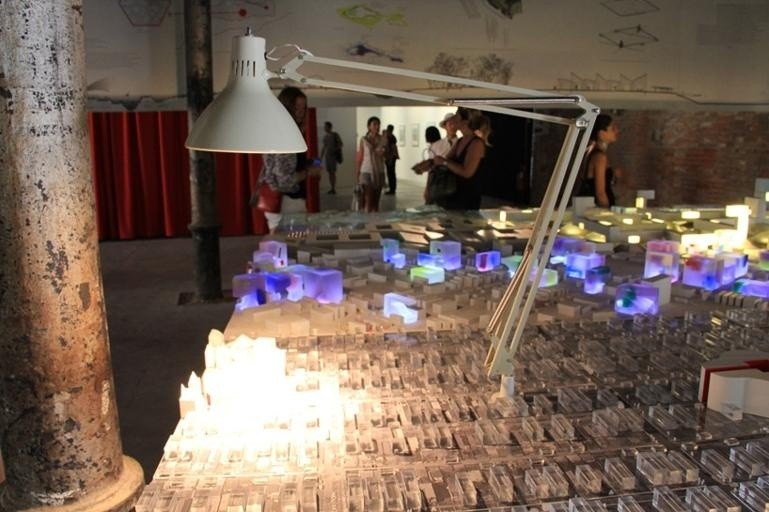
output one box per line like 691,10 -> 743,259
257,88 -> 324,235
428,113 -> 460,160
416,108 -> 488,210
474,115 -> 495,196
319,120 -> 343,194
425,125 -> 442,159
352,116 -> 390,213
578,114 -> 625,207
383,124 -> 400,195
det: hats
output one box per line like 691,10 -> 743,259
439,113 -> 457,127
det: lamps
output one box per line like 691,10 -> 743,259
183,29 -> 602,400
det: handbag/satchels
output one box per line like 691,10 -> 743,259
256,183 -> 281,213
335,148 -> 343,163
351,186 -> 365,211
423,165 -> 457,203
